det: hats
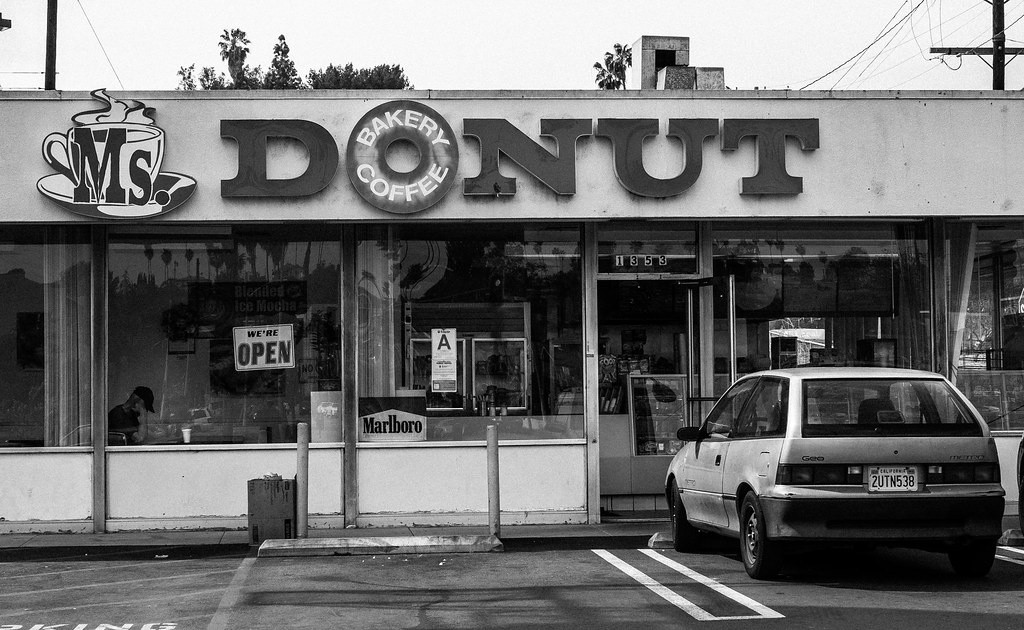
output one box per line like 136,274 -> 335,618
133,386 -> 156,413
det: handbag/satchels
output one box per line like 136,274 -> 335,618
545,351 -> 582,387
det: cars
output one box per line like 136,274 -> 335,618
168,407 -> 215,433
316,401 -> 339,417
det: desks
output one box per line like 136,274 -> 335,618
4,439 -> 44,445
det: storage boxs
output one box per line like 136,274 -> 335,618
247,471 -> 298,546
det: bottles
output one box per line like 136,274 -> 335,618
490,394 -> 495,417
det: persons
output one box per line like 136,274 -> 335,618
107,386 -> 155,445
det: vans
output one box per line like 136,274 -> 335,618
545,386 -> 583,439
663,367 -> 1006,580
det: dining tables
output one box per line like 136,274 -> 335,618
176,430 -> 246,445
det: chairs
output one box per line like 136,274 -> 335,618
857,397 -> 905,424
257,425 -> 273,443
108,432 -> 127,445
764,400 -> 788,435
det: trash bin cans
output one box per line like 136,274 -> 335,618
246,473 -> 297,548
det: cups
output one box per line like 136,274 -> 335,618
477,400 -> 486,415
500,405 -> 507,415
182,428 -> 192,443
